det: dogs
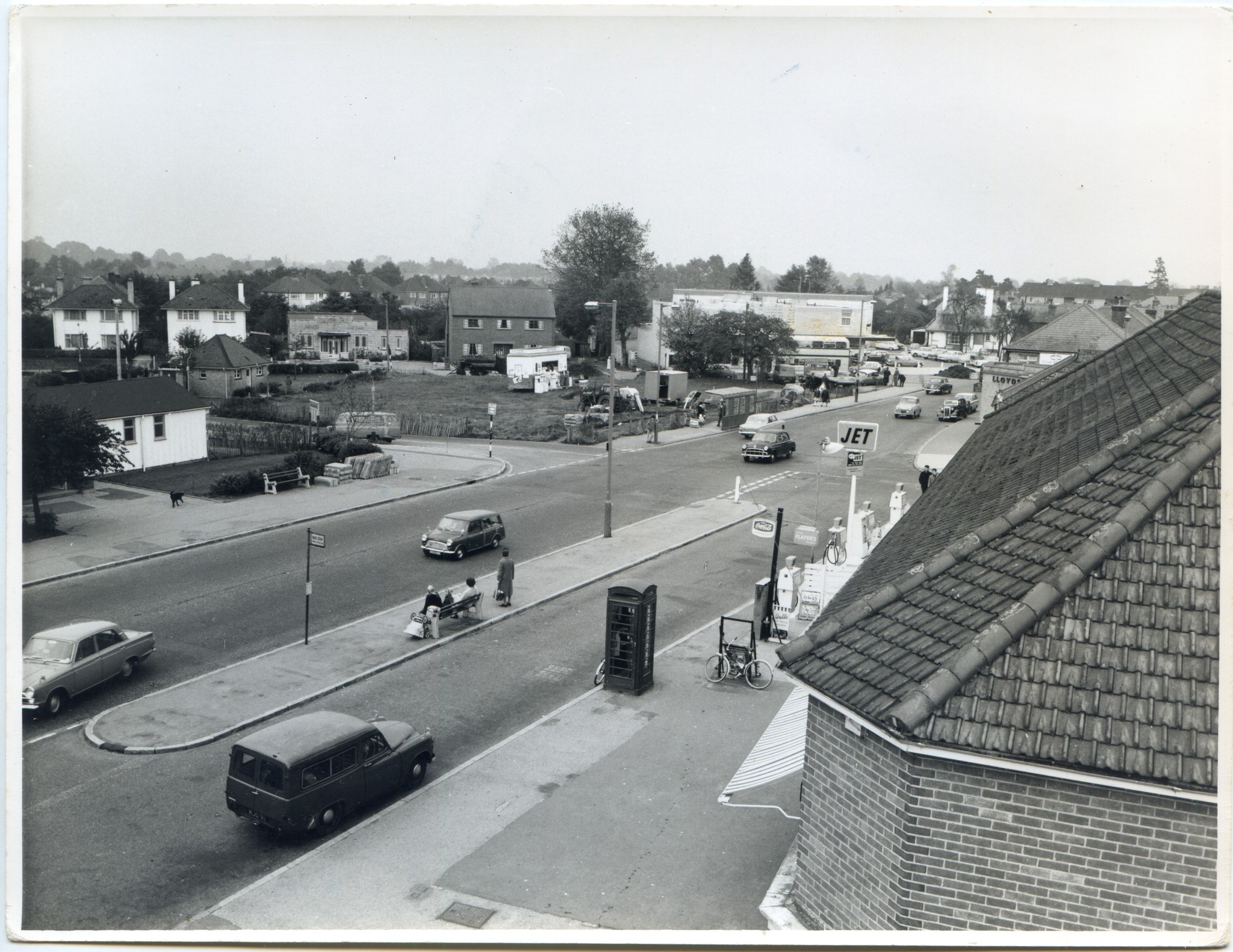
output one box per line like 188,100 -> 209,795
170,491 -> 185,508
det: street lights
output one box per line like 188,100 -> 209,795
854,300 -> 878,403
807,436 -> 844,593
584,299 -> 618,538
185,347 -> 192,392
368,363 -> 376,411
654,303 -> 682,442
379,300 -> 391,373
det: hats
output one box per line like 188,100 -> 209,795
448,589 -> 452,595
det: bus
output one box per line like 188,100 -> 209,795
334,411 -> 402,443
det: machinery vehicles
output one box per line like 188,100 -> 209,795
577,381 -> 626,413
772,363 -> 796,385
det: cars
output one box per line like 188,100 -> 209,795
848,334 -> 999,385
937,398 -> 968,422
738,413 -> 786,438
22,617 -> 157,718
894,396 -> 922,419
741,429 -> 796,463
924,377 -> 953,394
952,392 -> 980,414
705,364 -> 736,380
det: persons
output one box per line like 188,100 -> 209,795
450,576 -> 479,619
689,398 -> 726,427
927,468 -> 939,488
796,362 -> 839,407
442,589 -> 454,615
918,465 -> 932,494
497,548 -> 515,608
877,365 -> 906,387
419,585 -> 443,618
645,414 -> 656,443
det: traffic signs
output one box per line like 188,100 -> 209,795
310,532 -> 325,549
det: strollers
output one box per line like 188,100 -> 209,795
403,612 -> 430,639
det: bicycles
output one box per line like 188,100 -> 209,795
595,657 -> 605,685
704,633 -> 774,689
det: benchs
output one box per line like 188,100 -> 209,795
684,413 -> 705,425
420,590 -> 484,638
263,467 -> 310,495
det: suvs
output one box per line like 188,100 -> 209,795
223,709 -> 435,838
420,510 -> 506,562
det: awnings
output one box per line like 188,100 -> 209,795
717,686 -> 810,820
